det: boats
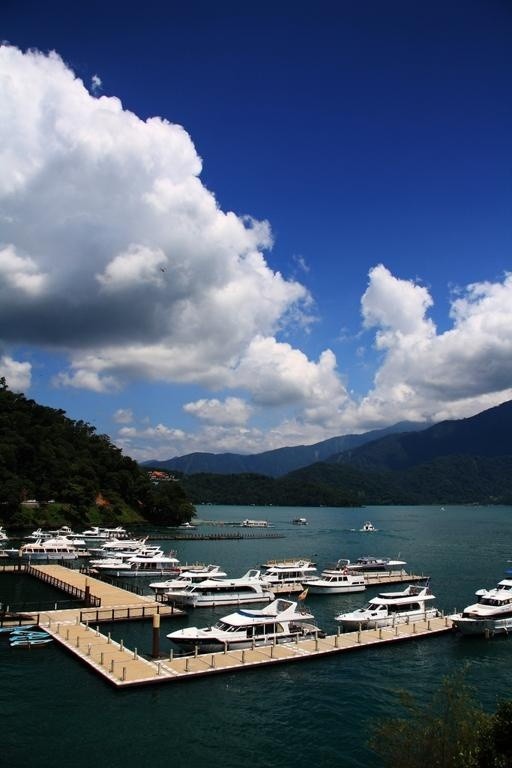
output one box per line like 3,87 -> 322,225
334,585 -> 442,629
166,598 -> 323,652
449,577 -> 512,636
0,624 -> 54,648
